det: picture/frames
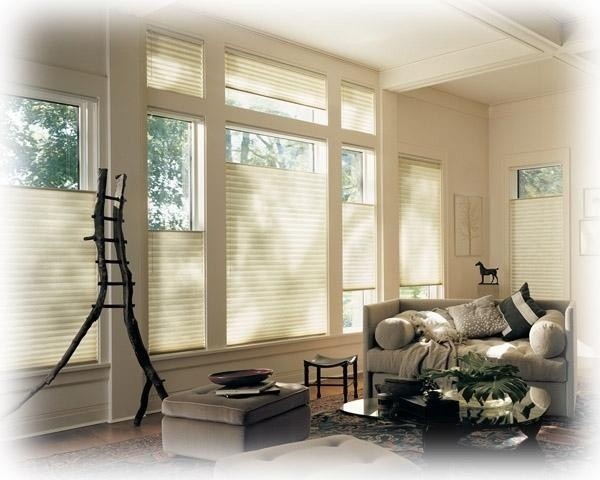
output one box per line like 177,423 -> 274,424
580,219 -> 600,257
467,195 -> 484,257
582,187 -> 600,218
454,193 -> 470,257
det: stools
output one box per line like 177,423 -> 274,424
161,380 -> 309,460
216,432 -> 424,465
303,353 -> 360,405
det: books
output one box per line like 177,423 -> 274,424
215,380 -> 276,394
222,384 -> 281,397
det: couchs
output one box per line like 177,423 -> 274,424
363,299 -> 576,420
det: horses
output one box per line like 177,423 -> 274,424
475,261 -> 499,284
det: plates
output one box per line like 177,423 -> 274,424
207,368 -> 273,386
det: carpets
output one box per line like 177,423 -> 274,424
27,394 -> 599,465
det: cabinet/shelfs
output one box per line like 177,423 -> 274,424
477,284 -> 499,300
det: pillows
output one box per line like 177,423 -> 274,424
530,309 -> 566,359
445,295 -> 507,338
375,309 -> 422,349
497,282 -> 545,343
410,307 -> 463,344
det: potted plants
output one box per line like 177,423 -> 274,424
422,351 -> 532,403
